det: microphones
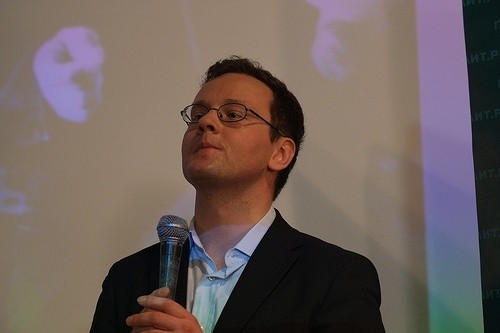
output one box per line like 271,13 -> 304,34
156,215 -> 190,300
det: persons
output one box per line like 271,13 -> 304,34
85,59 -> 386,333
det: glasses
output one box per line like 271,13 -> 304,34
181,103 -> 285,137
